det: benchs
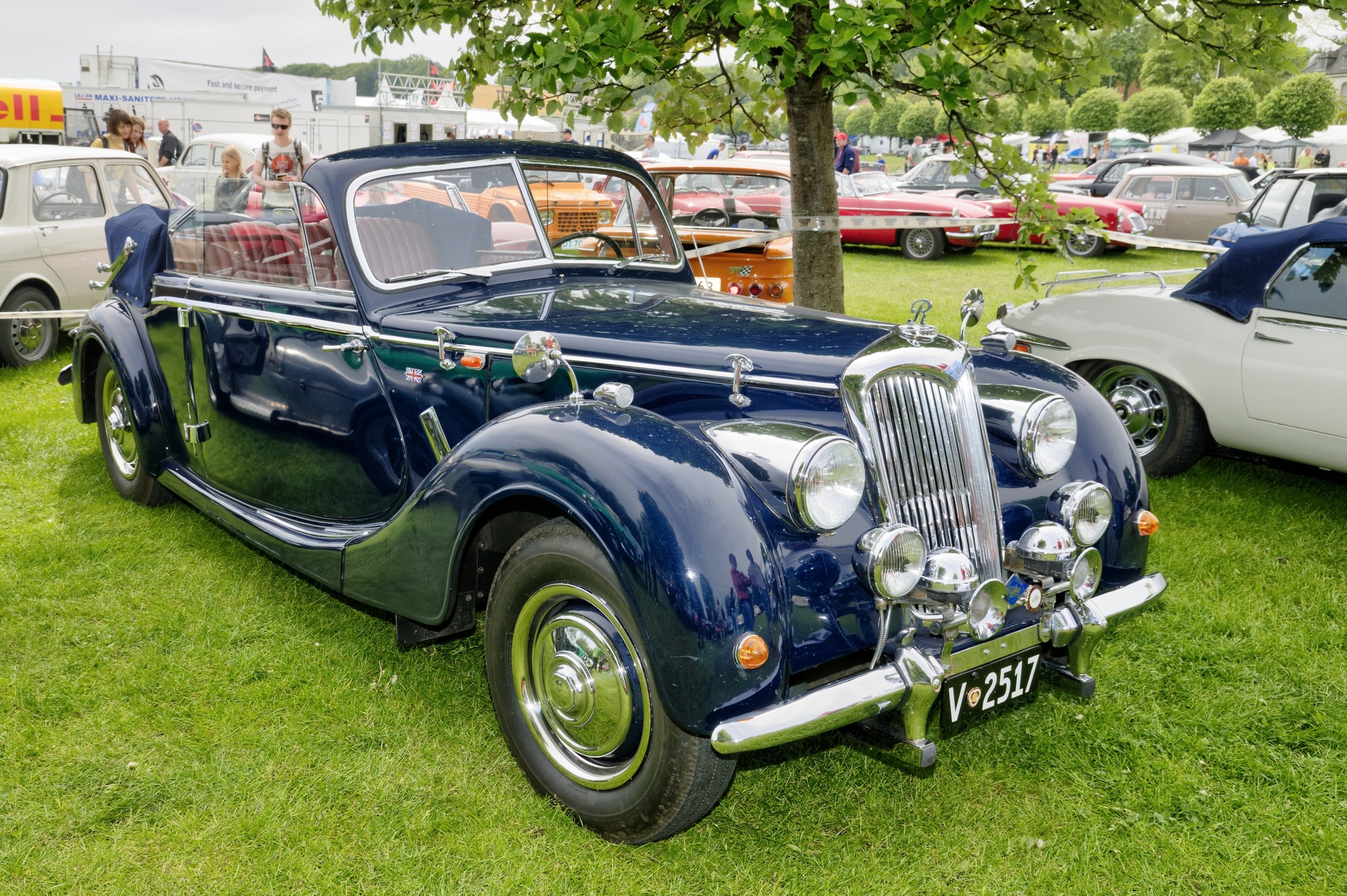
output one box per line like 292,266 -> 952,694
170,216 -> 440,291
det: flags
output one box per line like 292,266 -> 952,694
262,49 -> 276,72
429,60 -> 443,90
430,92 -> 437,105
442,82 -> 453,91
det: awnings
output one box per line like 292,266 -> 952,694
371,78 -> 558,141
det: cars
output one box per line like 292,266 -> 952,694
985,217 -> 1347,481
397,129 -> 1347,304
156,133 -> 275,204
1,143 -> 255,369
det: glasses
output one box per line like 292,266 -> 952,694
271,121 -> 290,130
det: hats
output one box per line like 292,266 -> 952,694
560,128 -> 572,134
834,132 -> 848,140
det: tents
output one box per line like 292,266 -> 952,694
937,126 -> 1347,168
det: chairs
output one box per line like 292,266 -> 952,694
1313,193 -> 1347,216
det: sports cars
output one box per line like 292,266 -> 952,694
57,139 -> 1170,846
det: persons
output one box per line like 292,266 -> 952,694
85,109 -> 142,204
118,116 -> 158,203
157,118 -> 178,167
559,130 -> 1330,175
213,145 -> 250,215
473,134 -> 532,140
446,132 -> 452,139
252,108 -> 313,216
